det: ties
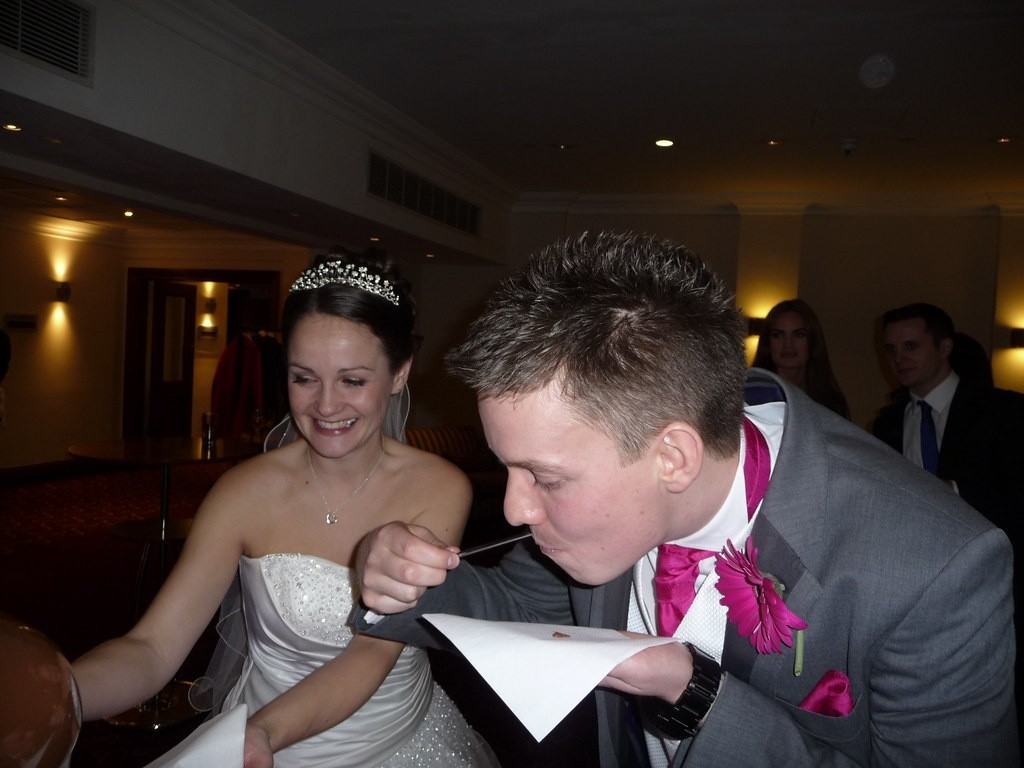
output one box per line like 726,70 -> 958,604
917,400 -> 939,472
653,415 -> 769,638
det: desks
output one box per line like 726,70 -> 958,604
101,683 -> 212,730
68,434 -> 260,625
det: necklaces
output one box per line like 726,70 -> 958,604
307,445 -> 386,523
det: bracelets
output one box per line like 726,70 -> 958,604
70,666 -> 84,726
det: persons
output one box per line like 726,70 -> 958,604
873,302 -> 1024,768
352,227 -> 1020,767
72,245 -> 500,768
945,331 -> 995,388
752,295 -> 852,424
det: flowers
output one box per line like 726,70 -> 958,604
715,537 -> 808,676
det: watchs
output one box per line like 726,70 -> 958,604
645,638 -> 724,737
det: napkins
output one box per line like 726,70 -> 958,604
424,615 -> 629,743
